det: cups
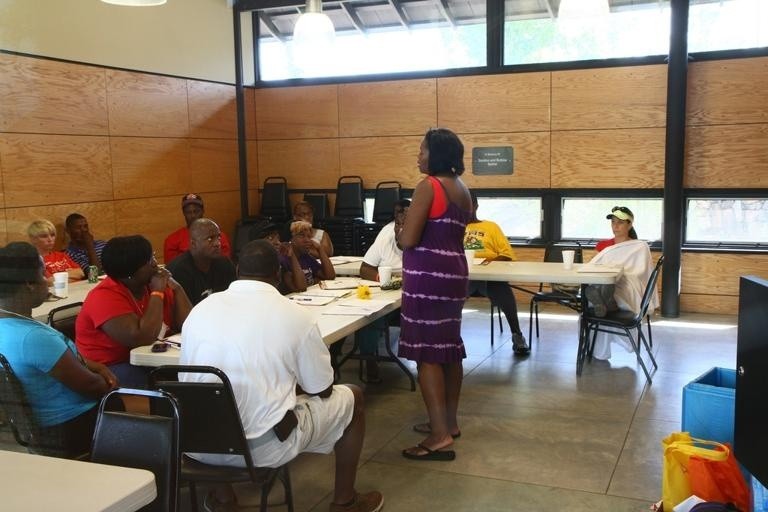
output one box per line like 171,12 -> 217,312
463,249 -> 475,273
561,249 -> 576,270
53,271 -> 68,298
377,266 -> 392,287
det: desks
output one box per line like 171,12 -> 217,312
119,286 -> 416,401
1,448 -> 156,511
32,263 -> 166,330
463,261 -> 620,373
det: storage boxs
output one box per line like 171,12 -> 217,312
676,368 -> 743,473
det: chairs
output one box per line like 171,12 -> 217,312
43,297 -> 84,339
147,351 -> 296,511
577,250 -> 668,376
250,167 -> 404,273
1,354 -> 88,458
522,237 -> 590,341
92,389 -> 184,512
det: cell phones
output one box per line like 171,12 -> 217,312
152,344 -> 168,352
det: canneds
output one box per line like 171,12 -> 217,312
88,266 -> 98,283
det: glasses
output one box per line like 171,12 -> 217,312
612,206 -> 633,216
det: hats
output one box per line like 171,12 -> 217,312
181,193 -> 203,207
248,219 -> 289,242
606,209 -> 633,223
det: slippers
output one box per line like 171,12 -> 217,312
412,419 -> 462,438
401,443 -> 456,463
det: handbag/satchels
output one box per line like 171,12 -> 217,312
662,431 -> 754,512
550,281 -> 580,308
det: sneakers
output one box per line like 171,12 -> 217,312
512,331 -> 530,355
329,488 -> 385,511
585,286 -> 607,318
601,280 -> 619,313
204,487 -> 243,512
365,359 -> 382,385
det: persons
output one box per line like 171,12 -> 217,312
166,217 -> 237,307
0,240 -> 127,447
60,212 -> 109,279
26,218 -> 85,288
291,199 -> 334,259
393,128 -> 474,453
164,192 -> 230,264
247,214 -> 307,298
75,233 -> 194,387
583,205 -> 638,319
359,199 -> 410,383
279,219 -> 335,287
177,237 -> 384,512
461,189 -> 530,356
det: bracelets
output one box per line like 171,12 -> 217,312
149,289 -> 164,300
396,226 -> 403,240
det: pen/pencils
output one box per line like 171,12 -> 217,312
289,297 -> 312,301
165,340 -> 181,348
